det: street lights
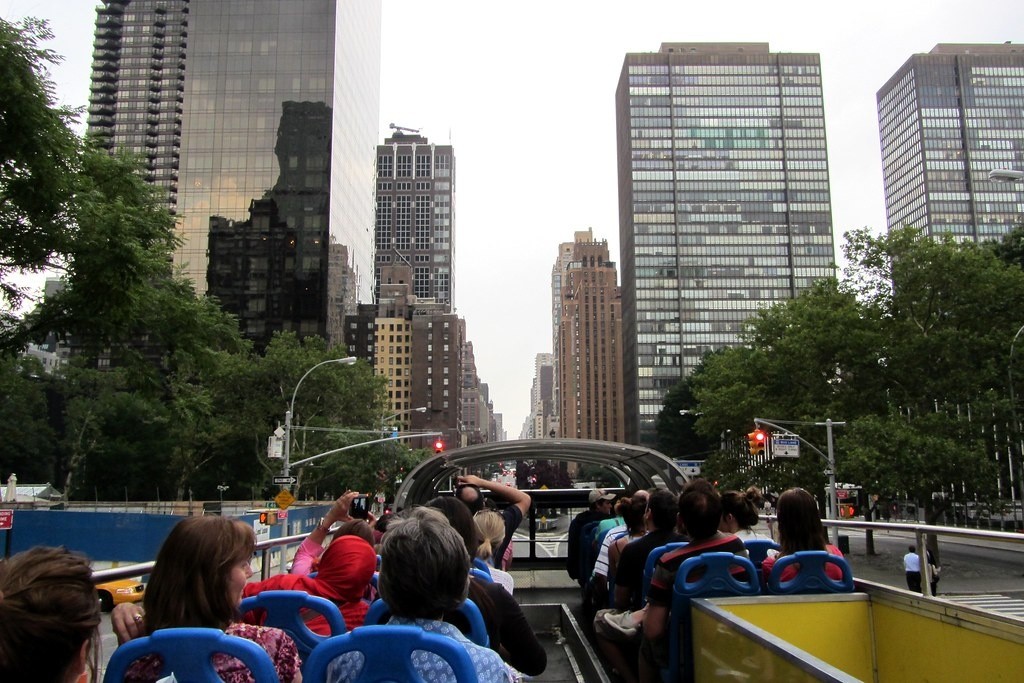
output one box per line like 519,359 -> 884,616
279,357 -> 357,575
380,406 -> 427,469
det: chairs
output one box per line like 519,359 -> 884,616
590,520 -> 856,683
103,554 -> 495,683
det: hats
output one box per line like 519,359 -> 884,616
589,490 -> 616,503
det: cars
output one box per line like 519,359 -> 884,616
95,579 -> 147,613
490,467 -> 517,489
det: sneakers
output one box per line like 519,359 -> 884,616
604,609 -> 637,635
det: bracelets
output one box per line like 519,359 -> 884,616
318,525 -> 329,533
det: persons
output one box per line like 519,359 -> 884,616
927,549 -> 940,597
0,474 -> 547,683
903,545 -> 922,593
566,479 -> 846,683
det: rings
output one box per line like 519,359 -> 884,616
133,616 -> 140,620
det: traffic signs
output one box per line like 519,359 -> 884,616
272,475 -> 298,486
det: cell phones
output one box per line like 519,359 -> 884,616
349,494 -> 369,520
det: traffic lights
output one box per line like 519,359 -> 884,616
747,432 -> 757,455
259,512 -> 268,524
435,441 -> 442,454
550,430 -> 556,438
714,481 -> 719,486
755,430 -> 765,455
